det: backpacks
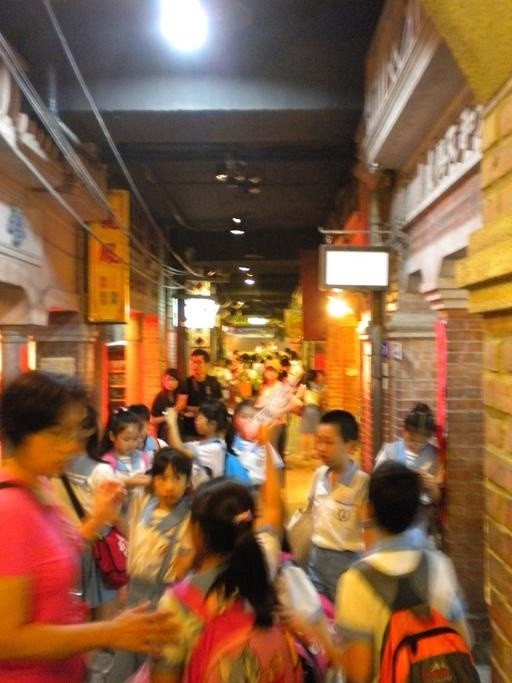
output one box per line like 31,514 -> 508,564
174,552 -> 326,683
352,550 -> 480,683
204,440 -> 251,487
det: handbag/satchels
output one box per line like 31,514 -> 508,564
93,527 -> 128,589
